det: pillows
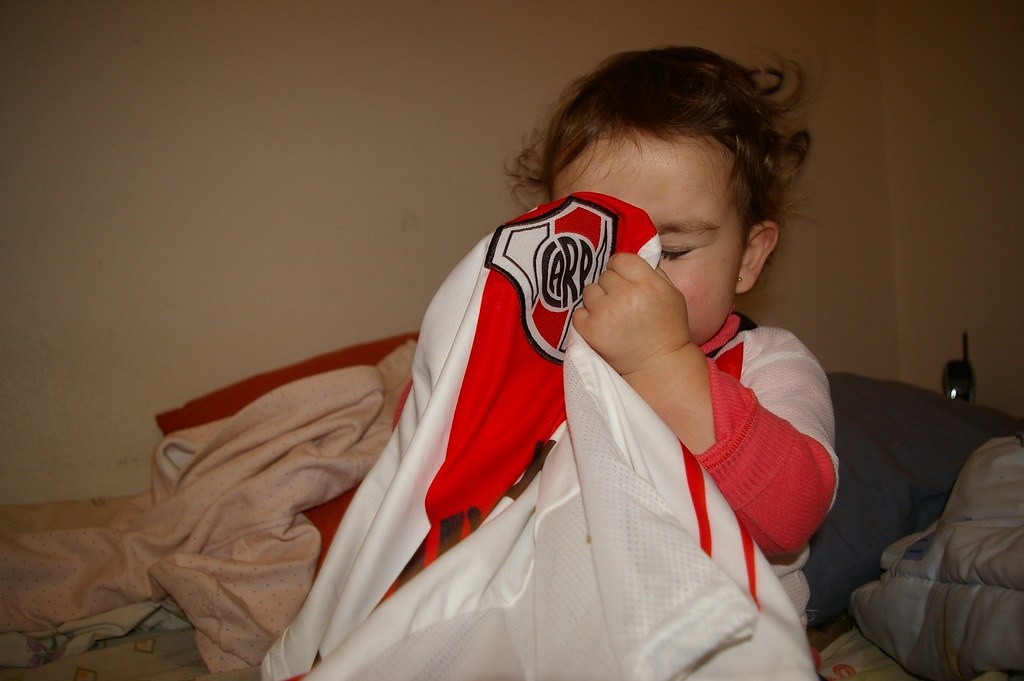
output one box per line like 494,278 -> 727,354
800,367 -> 1024,626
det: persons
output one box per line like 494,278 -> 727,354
260,45 -> 837,681
